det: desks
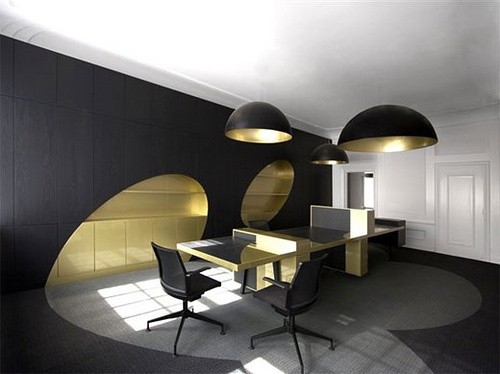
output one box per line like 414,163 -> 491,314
176,219 -> 406,294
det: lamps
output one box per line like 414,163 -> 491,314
223,102 -> 294,144
337,105 -> 439,151
309,144 -> 349,166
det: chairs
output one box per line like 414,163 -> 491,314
248,253 -> 337,373
143,241 -> 226,356
